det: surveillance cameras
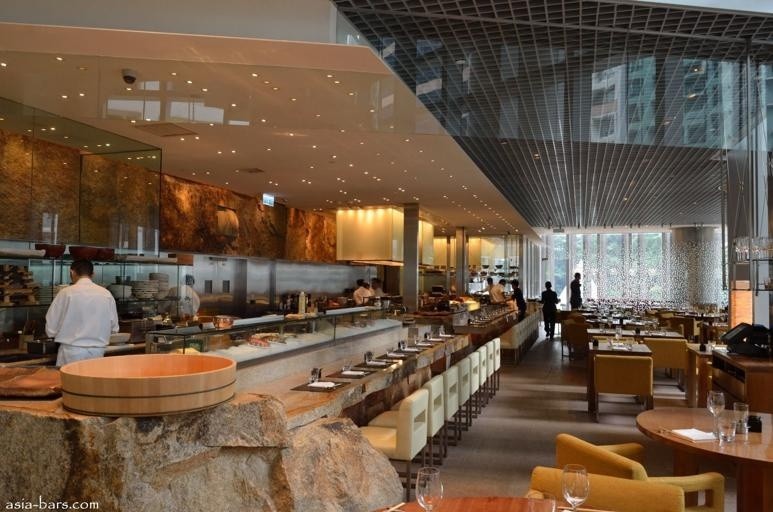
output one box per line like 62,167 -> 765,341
122,70 -> 138,85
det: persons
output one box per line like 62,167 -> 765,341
535,281 -> 561,340
505,279 -> 526,321
476,276 -> 495,294
41,257 -> 120,365
371,279 -> 382,295
570,273 -> 582,309
362,283 -> 374,295
353,279 -> 368,305
158,271 -> 199,316
490,279 -> 508,305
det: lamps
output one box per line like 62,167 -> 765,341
334,206 -> 435,268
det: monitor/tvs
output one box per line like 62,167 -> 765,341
720,322 -> 750,346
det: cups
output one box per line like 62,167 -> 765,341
342,358 -> 352,371
427,332 -> 432,339
439,328 -> 445,335
526,492 -> 556,511
386,346 -> 393,356
308,372 -> 318,384
722,418 -> 736,443
733,402 -> 748,433
365,354 -> 374,365
399,339 -> 407,350
598,315 -> 667,350
413,335 -> 420,345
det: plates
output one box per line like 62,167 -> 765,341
35,273 -> 169,302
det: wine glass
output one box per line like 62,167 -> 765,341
734,235 -> 773,260
561,463 -> 589,511
415,466 -> 443,511
707,391 -> 726,441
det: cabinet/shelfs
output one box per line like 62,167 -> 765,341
0,249 -> 182,369
141,304 -> 405,400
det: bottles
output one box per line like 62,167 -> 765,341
298,292 -> 305,314
749,414 -> 762,433
152,312 -> 171,331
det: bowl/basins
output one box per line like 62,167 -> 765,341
98,248 -> 115,260
469,264 -> 504,276
212,316 -> 233,330
110,332 -> 131,344
58,351 -> 237,416
34,243 -> 64,260
68,245 -> 96,260
